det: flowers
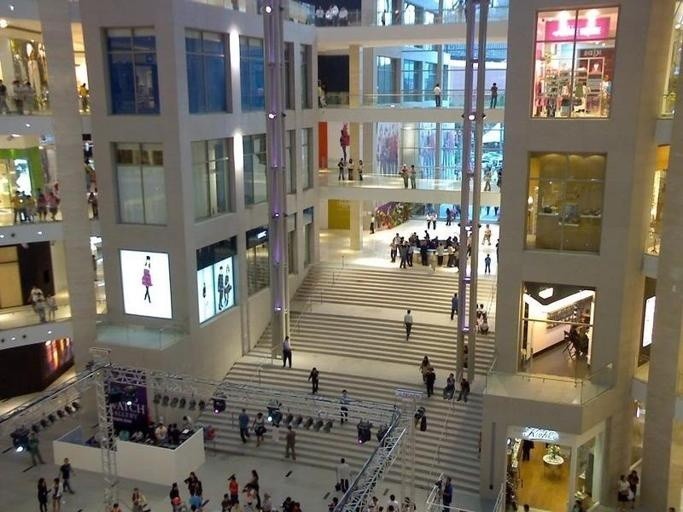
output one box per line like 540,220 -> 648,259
546,444 -> 560,461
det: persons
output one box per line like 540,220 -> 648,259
9,182 -> 98,224
390,206 -> 499,274
338,157 -> 363,181
434,84 -> 441,107
490,83 -> 498,108
484,164 -> 503,190
400,164 -> 417,189
218,265 -> 233,309
282,336 -> 293,368
315,3 -> 348,26
0,79 -> 89,115
600,75 -> 611,96
26,366 -> 418,512
382,9 -> 387,25
404,292 -> 489,402
317,82 -> 327,108
93,255 -> 98,281
28,287 -> 57,323
370,214 -> 375,234
439,440 -> 640,512
141,256 -> 153,303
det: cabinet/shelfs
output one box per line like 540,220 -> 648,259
545,57 -> 606,119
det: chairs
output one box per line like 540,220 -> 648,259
563,329 -> 588,362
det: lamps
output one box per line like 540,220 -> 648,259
12,400 -> 97,451
263,410 -> 333,434
151,396 -> 209,413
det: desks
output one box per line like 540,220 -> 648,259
542,454 -> 564,481
